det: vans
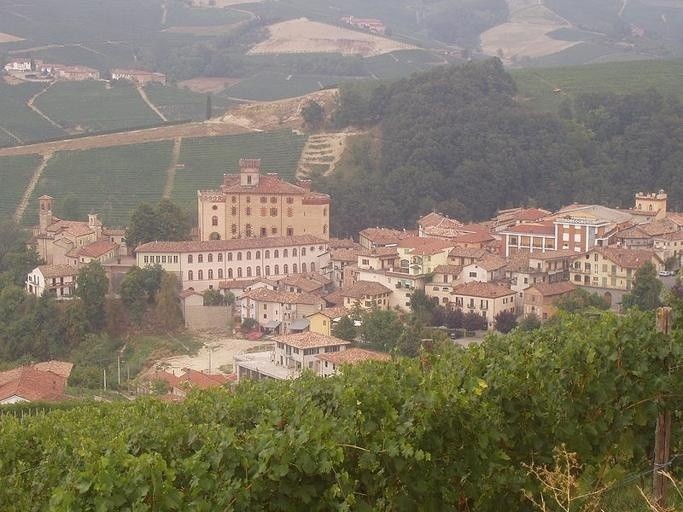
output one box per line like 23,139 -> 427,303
659,270 -> 675,277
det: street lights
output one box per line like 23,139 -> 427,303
115,349 -> 125,389
203,343 -> 219,377
